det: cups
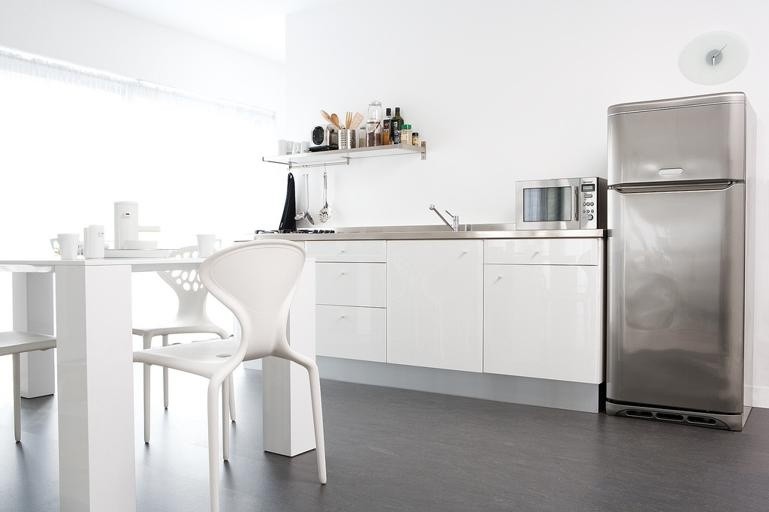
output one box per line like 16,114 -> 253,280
196,234 -> 222,259
50,232 -> 80,260
83,227 -> 105,259
338,129 -> 356,150
278,139 -> 310,155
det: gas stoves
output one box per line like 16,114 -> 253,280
255,229 -> 338,234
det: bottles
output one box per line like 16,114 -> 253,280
359,100 -> 419,147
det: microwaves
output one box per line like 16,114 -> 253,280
513,176 -> 607,230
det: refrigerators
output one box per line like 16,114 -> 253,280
605,91 -> 757,432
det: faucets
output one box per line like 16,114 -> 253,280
428,204 -> 459,232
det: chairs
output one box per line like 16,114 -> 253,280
133,245 -> 236,422
133,239 -> 327,512
0,330 -> 56,442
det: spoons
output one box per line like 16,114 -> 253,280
331,114 -> 340,130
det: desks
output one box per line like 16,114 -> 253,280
0,259 -> 230,512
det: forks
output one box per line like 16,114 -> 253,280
346,112 -> 352,129
